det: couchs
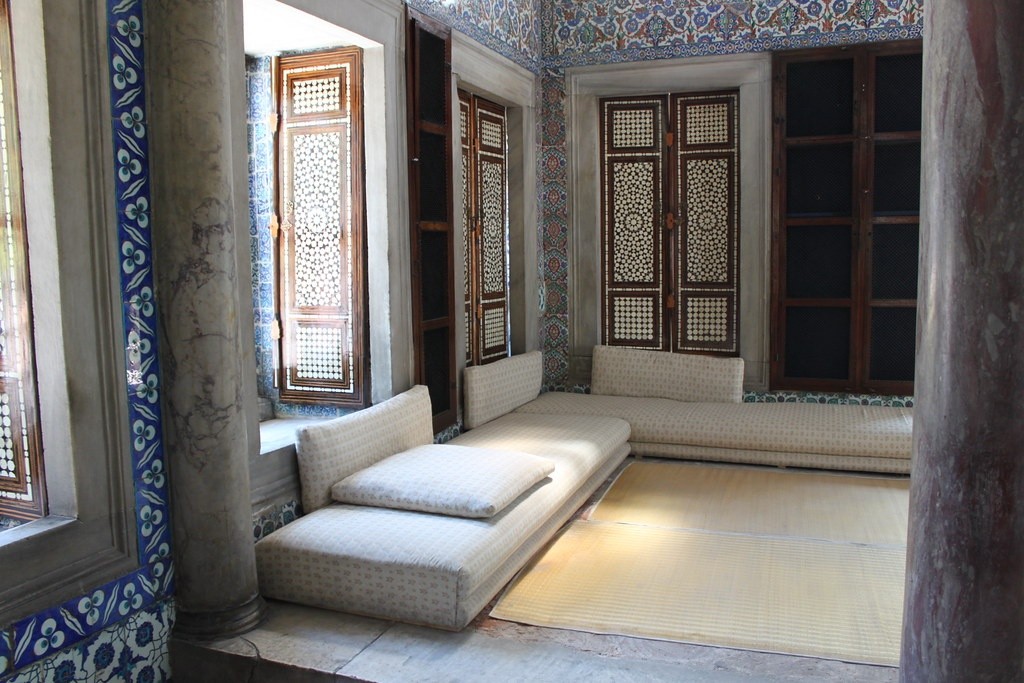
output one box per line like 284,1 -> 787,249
254,414 -> 631,632
513,392 -> 913,476
